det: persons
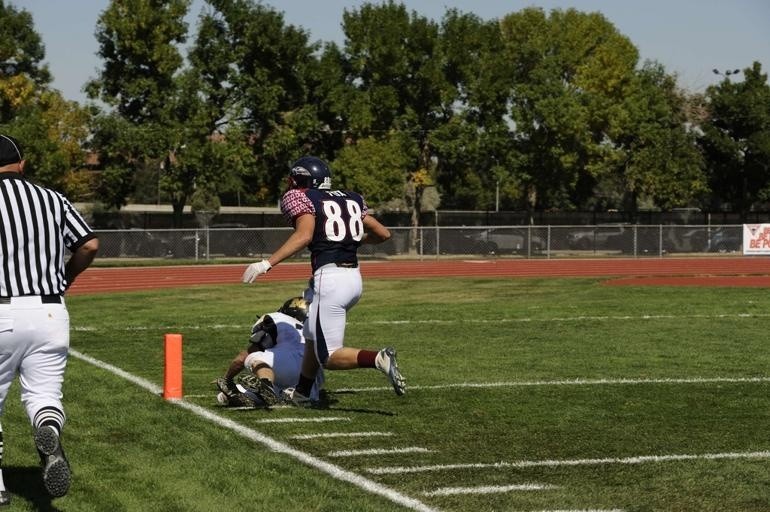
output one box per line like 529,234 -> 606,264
237,297 -> 311,410
0,133 -> 100,504
213,343 -> 264,406
240,157 -> 409,407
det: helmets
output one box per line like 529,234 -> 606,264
280,296 -> 309,320
288,157 -> 334,190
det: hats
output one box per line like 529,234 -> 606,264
1,134 -> 24,166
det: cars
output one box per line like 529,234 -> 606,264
103,227 -> 169,257
459,225 -> 553,257
656,225 -> 743,253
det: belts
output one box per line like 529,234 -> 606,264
0,295 -> 63,305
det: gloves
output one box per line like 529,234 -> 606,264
243,260 -> 271,284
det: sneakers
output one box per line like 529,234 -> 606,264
238,373 -> 276,405
217,376 -> 247,406
280,386 -> 312,407
375,347 -> 407,395
36,421 -> 71,498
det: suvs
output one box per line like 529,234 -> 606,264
565,223 -> 638,249
172,223 -> 266,257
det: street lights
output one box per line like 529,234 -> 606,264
711,66 -> 741,84
